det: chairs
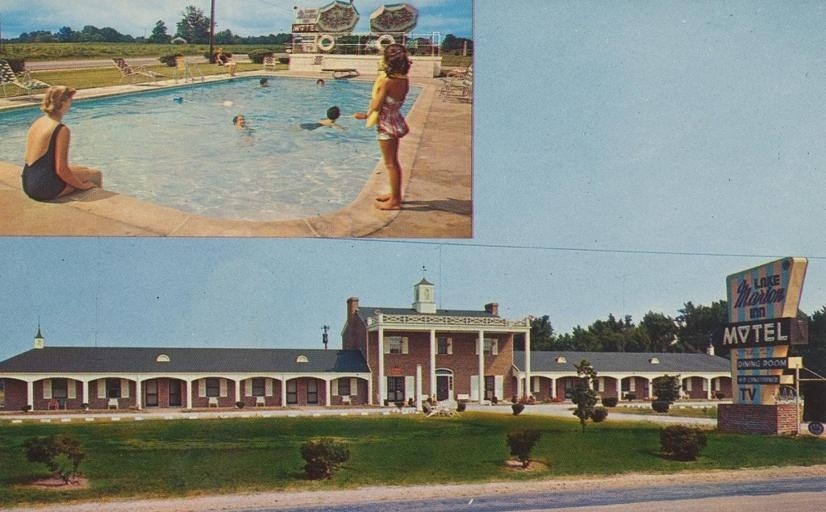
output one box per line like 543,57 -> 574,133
320,68 -> 360,80
211,54 -> 232,78
262,51 -> 278,72
1,55 -> 53,102
310,56 -> 322,70
439,62 -> 471,105
111,55 -> 171,88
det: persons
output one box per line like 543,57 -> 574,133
316,79 -> 325,88
257,78 -> 270,88
233,114 -> 248,130
430,401 -> 439,410
318,106 -> 344,131
21,86 -> 102,201
353,44 -> 410,210
212,52 -> 223,65
